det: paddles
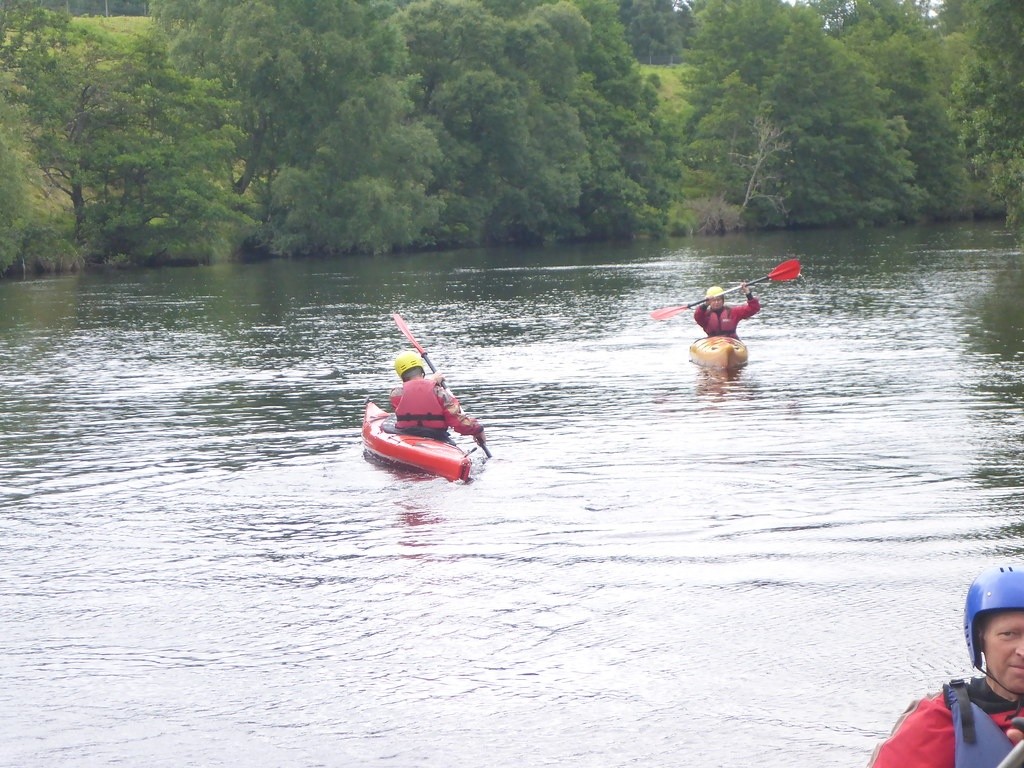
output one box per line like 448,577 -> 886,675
392,312 -> 493,458
650,259 -> 801,321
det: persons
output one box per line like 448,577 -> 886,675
694,283 -> 760,340
867,565 -> 1024,768
389,351 -> 487,447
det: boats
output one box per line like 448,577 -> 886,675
361,402 -> 477,484
690,333 -> 749,371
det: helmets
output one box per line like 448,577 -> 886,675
706,286 -> 723,298
964,562 -> 1024,667
395,351 -> 423,377
